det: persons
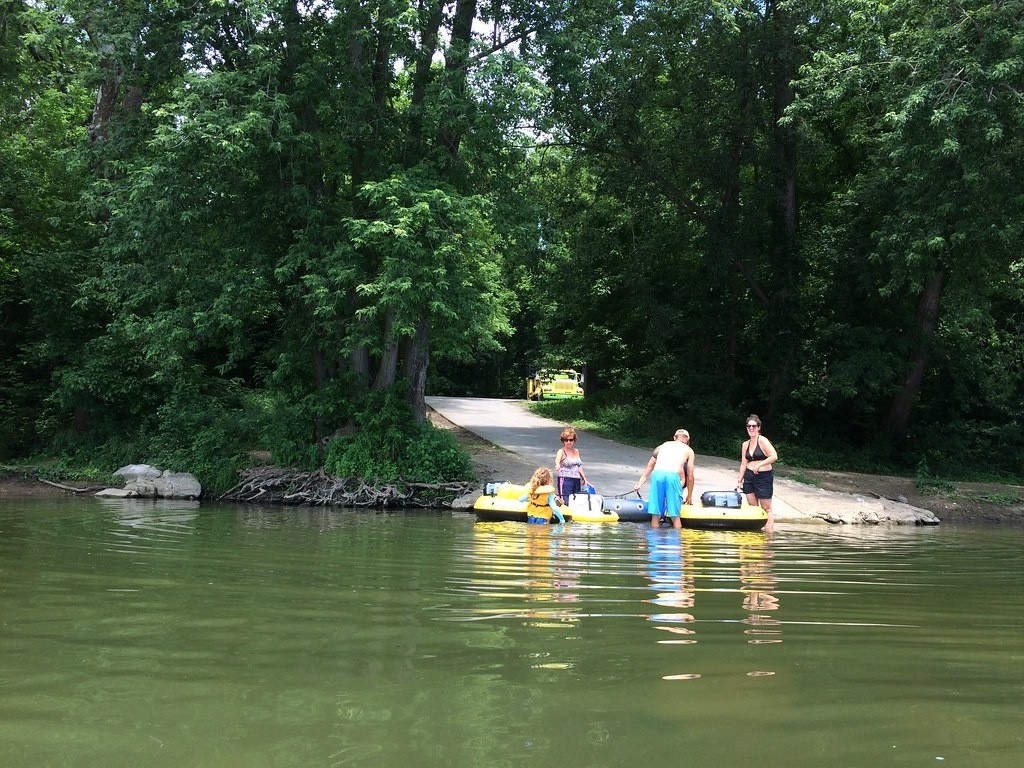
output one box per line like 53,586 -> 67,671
737,413 -> 778,531
519,467 -> 565,525
634,429 -> 694,528
554,427 -> 587,507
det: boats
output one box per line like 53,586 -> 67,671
472,480 -> 620,524
564,481 -> 652,525
665,487 -> 768,530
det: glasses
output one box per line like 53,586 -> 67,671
747,425 -> 758,428
564,439 -> 574,442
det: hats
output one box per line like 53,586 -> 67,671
675,429 -> 689,437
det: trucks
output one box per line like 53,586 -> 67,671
526,368 -> 584,401
